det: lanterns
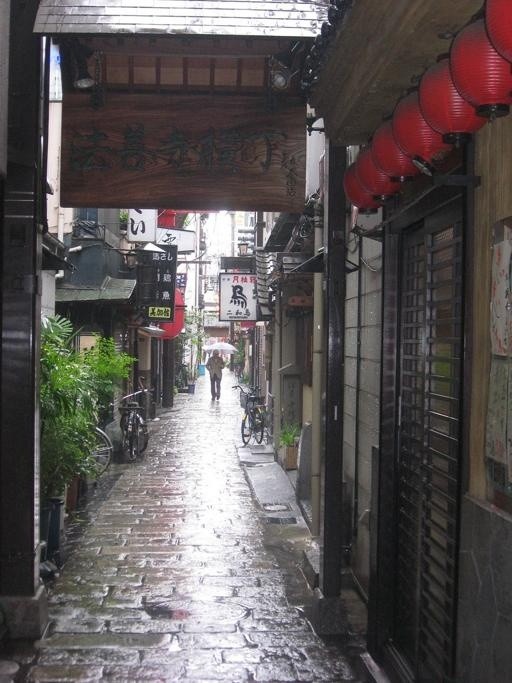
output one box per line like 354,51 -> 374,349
419,59 -> 486,144
355,146 -> 403,202
448,13 -> 512,120
160,289 -> 184,343
390,90 -> 456,175
343,169 -> 385,208
483,0 -> 512,63
371,120 -> 422,179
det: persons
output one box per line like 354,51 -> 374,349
205,351 -> 226,400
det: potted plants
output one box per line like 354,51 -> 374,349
279,420 -> 301,469
38,315 -> 138,564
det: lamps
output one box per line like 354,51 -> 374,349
66,38 -> 95,89
237,236 -> 255,256
264,40 -> 305,95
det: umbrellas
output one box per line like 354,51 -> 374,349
202,339 -> 238,364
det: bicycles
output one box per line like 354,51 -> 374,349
118,399 -> 149,461
231,383 -> 267,444
